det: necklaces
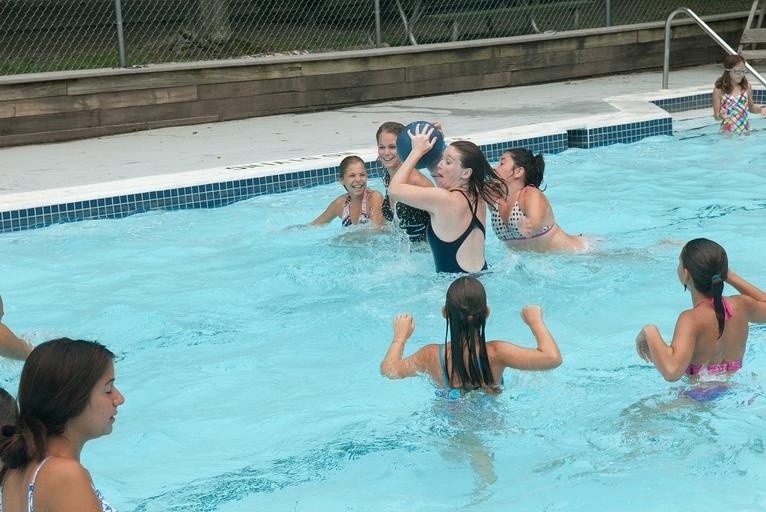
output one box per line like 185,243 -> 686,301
732,87 -> 743,91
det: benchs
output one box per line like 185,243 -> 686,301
424,0 -> 597,42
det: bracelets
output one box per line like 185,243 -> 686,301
391,339 -> 405,346
717,113 -> 721,121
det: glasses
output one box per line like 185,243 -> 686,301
724,66 -> 750,75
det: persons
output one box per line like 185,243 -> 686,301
635,236 -> 766,384
0,335 -> 125,512
387,123 -> 512,274
428,402 -> 517,496
381,275 -> 564,400
623,385 -> 763,448
425,119 -> 592,258
283,154 -> 386,248
0,296 -> 33,363
712,55 -> 765,138
375,121 -> 434,244
0,384 -> 19,460
